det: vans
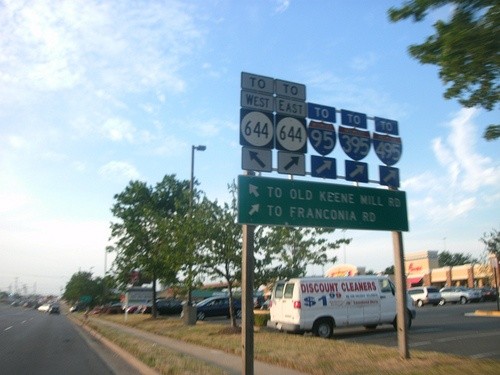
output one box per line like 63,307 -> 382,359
267,274 -> 417,340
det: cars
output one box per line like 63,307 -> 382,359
408,286 -> 442,307
8,293 -> 60,314
68,289 -> 272,321
438,284 -> 499,305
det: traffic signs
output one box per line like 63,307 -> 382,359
237,174 -> 410,232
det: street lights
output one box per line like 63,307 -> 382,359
186,144 -> 207,304
